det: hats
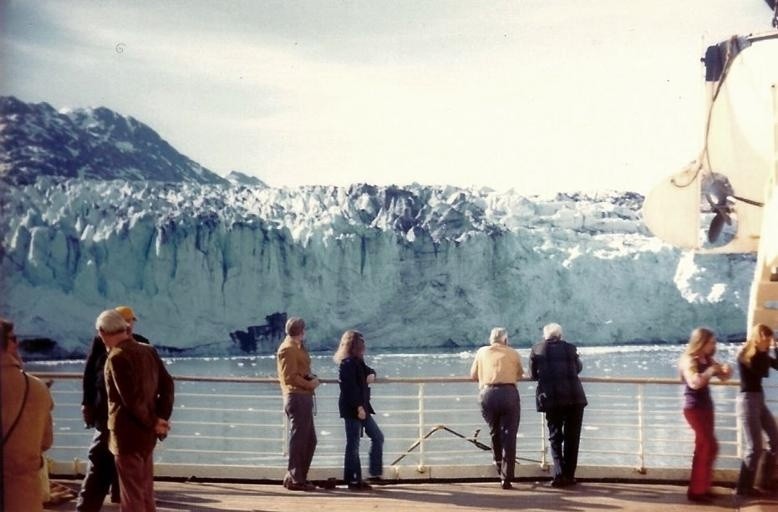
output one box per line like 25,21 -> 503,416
116,306 -> 139,321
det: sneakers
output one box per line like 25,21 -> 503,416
552,478 -> 579,486
688,492 -> 721,505
737,488 -> 762,497
349,478 -> 391,491
283,475 -> 317,490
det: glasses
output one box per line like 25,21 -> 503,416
7,335 -> 18,344
359,339 -> 366,343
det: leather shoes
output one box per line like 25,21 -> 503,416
493,461 -> 512,488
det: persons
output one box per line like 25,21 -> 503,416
679,328 -> 732,504
734,324 -> 778,498
529,322 -> 588,488
96,310 -> 175,512
75,307 -> 150,512
0,321 -> 55,512
277,317 -> 320,491
334,330 -> 387,491
471,327 -> 524,490
